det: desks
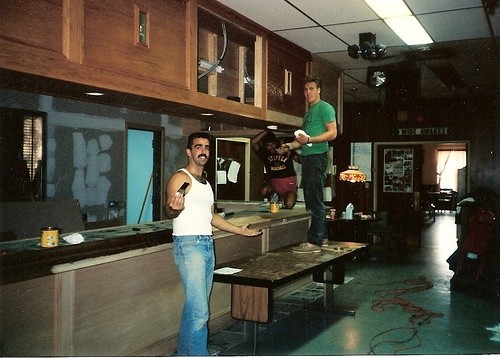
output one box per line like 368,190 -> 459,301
326,215 -> 382,243
212,241 -> 368,356
424,191 -> 457,212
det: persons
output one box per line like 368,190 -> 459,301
165,131 -> 263,356
251,128 -> 302,209
282,74 -> 337,254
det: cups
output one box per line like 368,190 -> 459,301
40,226 -> 59,248
270,201 -> 279,213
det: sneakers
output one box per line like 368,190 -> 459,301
290,242 -> 322,254
321,240 -> 329,247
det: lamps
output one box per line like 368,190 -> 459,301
348,32 -> 392,61
340,89 -> 368,183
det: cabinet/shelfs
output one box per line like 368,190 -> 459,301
264,39 -> 311,126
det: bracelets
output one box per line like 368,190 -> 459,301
308,135 -> 311,144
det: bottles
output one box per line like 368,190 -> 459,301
345,202 -> 355,220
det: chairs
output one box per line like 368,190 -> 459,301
342,201 -> 408,261
421,188 -> 453,222
